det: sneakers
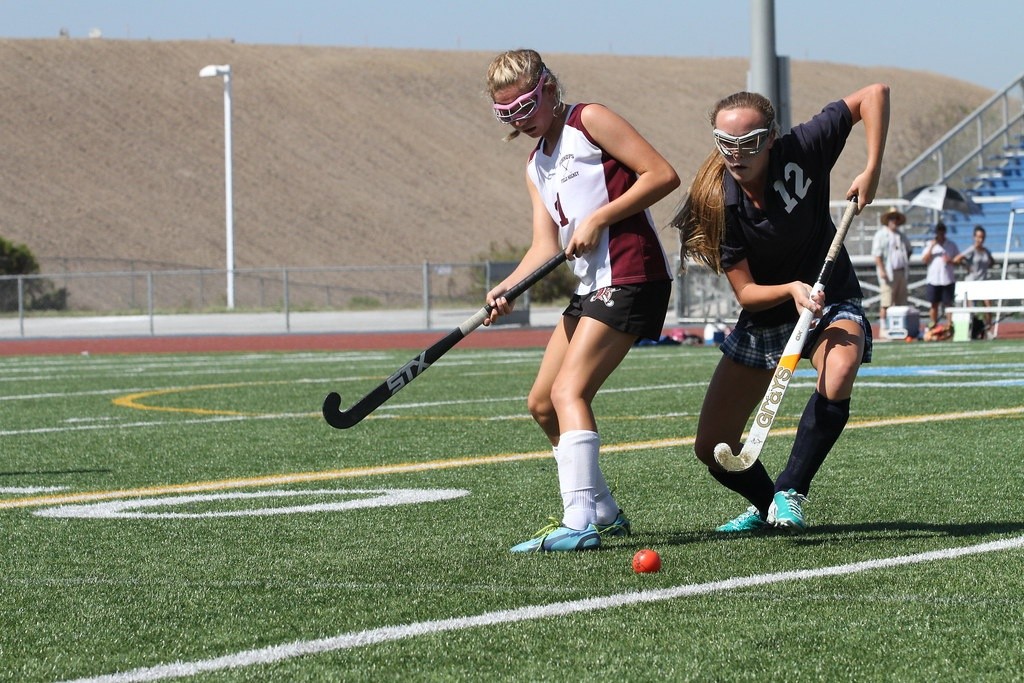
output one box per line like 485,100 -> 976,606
508,516 -> 601,551
595,509 -> 631,539
715,505 -> 768,538
766,488 -> 811,533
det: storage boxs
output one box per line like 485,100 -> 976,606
886,305 -> 920,337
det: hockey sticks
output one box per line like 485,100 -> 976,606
714,196 -> 858,474
321,245 -> 578,431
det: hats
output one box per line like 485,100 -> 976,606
880,207 -> 907,226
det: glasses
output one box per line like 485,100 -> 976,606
713,124 -> 777,159
493,67 -> 548,124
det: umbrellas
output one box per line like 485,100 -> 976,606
899,183 -> 987,225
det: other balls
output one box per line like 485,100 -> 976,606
631,549 -> 661,574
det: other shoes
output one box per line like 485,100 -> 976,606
984,330 -> 996,341
879,328 -> 886,337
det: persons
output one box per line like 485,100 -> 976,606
951,225 -> 996,331
870,206 -> 913,339
668,78 -> 891,537
920,222 -> 961,333
482,50 -> 681,553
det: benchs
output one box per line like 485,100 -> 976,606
908,136 -> 1024,253
945,280 -> 1024,342
